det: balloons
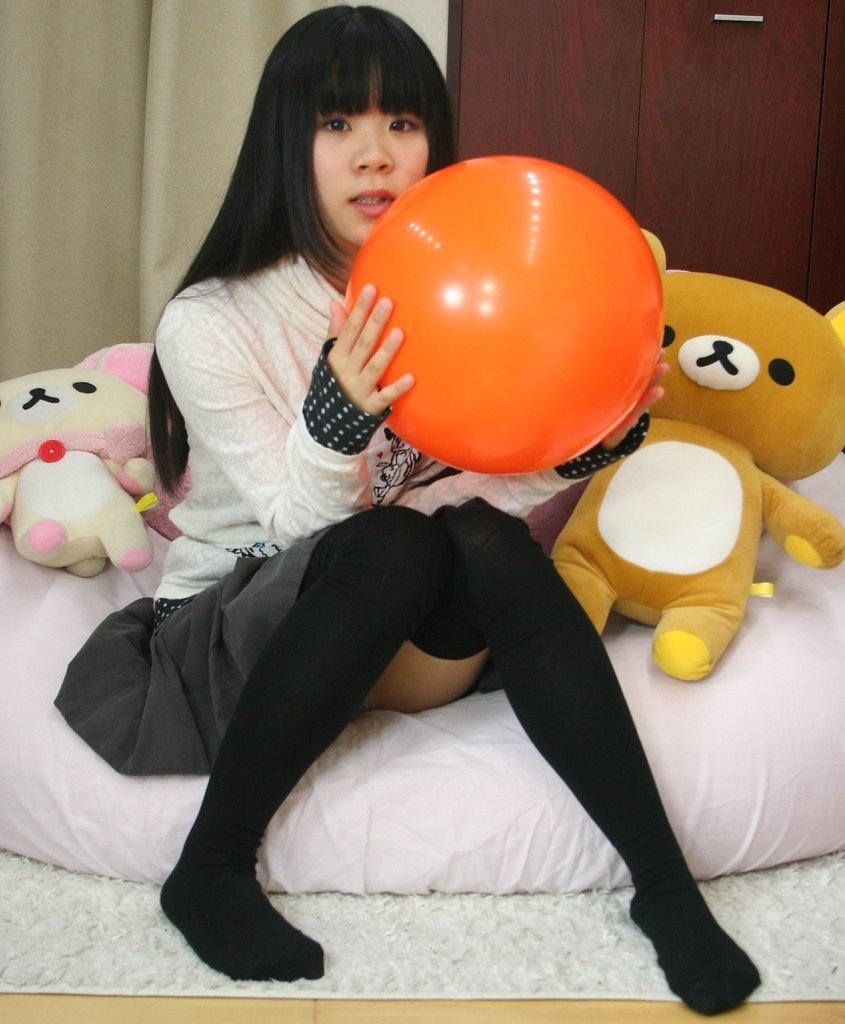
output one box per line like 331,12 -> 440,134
344,153 -> 664,476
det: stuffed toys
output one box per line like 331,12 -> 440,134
550,225 -> 845,682
0,343 -> 191,580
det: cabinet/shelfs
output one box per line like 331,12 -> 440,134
446,0 -> 845,316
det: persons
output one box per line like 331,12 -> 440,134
54,2 -> 756,1018
